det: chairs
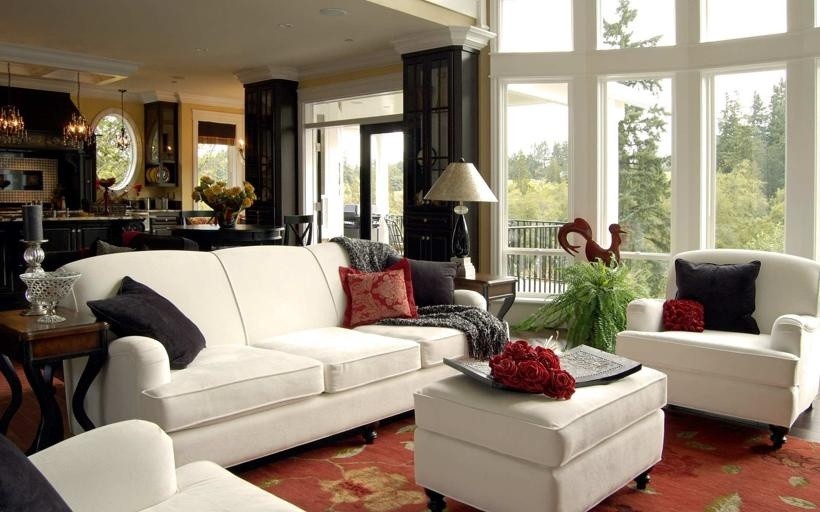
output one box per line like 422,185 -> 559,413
613,248 -> 820,454
23,417 -> 307,510
282,213 -> 314,247
384,212 -> 409,256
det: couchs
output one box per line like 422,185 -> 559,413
49,239 -> 491,474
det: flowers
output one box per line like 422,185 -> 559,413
663,297 -> 706,333
190,174 -> 259,213
488,339 -> 580,403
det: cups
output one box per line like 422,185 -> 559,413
144,197 -> 151,210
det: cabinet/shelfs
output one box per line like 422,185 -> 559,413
14,221 -> 76,293
149,210 -> 183,234
77,222 -> 111,258
1,220 -> 13,296
144,100 -> 181,188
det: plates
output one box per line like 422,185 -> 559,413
146,166 -> 170,184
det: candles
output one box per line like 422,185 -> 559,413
20,202 -> 45,242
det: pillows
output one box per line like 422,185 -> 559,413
86,275 -> 208,373
0,432 -> 74,510
384,254 -> 459,306
673,256 -> 768,335
337,254 -> 418,329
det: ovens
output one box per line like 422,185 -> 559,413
151,216 -> 181,234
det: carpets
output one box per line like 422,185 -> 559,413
222,397 -> 818,512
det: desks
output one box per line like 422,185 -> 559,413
1,302 -> 112,457
171,223 -> 287,252
340,204 -> 383,242
453,271 -> 519,323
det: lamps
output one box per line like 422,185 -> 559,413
0,59 -> 33,147
60,71 -> 99,150
422,157 -> 504,280
113,87 -> 134,151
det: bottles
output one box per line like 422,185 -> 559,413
61,196 -> 66,209
155,196 -> 168,210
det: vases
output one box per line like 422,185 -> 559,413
214,207 -> 241,228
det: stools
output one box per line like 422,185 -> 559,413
411,361 -> 670,510
